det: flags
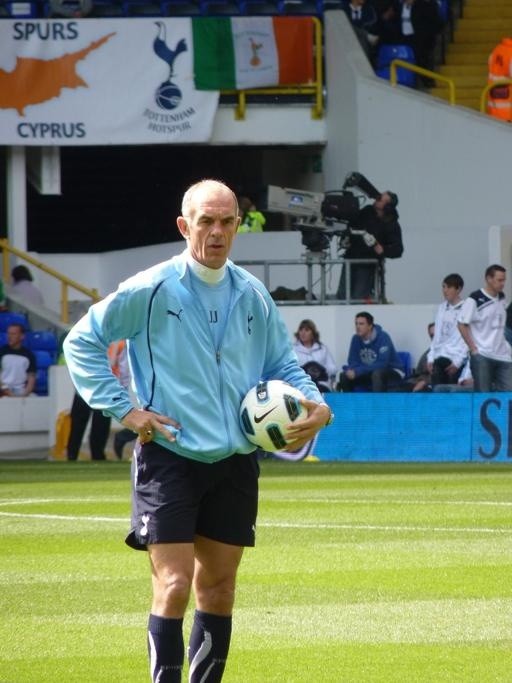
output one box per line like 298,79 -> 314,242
191,15 -> 316,91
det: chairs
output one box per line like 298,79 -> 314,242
342,352 -> 411,391
0,313 -> 60,396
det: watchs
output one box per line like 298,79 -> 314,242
321,403 -> 335,426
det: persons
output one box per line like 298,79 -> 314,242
487,36 -> 512,121
412,320 -> 436,392
338,310 -> 407,391
56,172 -> 337,683
379,0 -> 447,87
426,272 -> 470,393
8,264 -> 45,304
47,0 -> 96,17
0,323 -> 37,397
456,263 -> 511,393
341,0 -> 380,55
64,390 -> 113,462
291,319 -> 339,391
233,194 -> 267,232
334,188 -> 405,299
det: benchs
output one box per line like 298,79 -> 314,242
374,44 -> 417,92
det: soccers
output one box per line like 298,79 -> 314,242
240,381 -> 311,453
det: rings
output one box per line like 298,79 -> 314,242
146,428 -> 153,435
